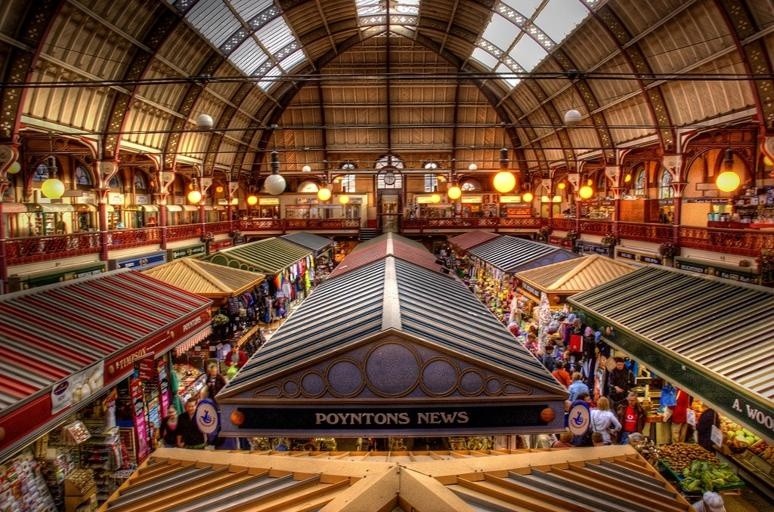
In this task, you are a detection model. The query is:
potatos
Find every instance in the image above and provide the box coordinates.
[652,442,720,473]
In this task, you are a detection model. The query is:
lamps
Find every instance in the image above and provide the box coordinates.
[41,153,65,200]
[432,177,440,202]
[557,171,631,198]
[247,190,257,205]
[564,81,583,128]
[196,82,214,129]
[492,161,517,194]
[264,132,287,196]
[524,176,533,202]
[317,180,331,200]
[189,172,201,204]
[303,150,312,173]
[447,180,462,199]
[298,180,317,193]
[716,147,741,193]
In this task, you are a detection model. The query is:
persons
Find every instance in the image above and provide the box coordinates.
[285,202,613,219]
[707,211,739,223]
[658,205,674,223]
[232,209,279,220]
[54,216,143,232]
[691,489,727,512]
[432,241,720,452]
[159,239,354,450]
[147,211,227,227]
